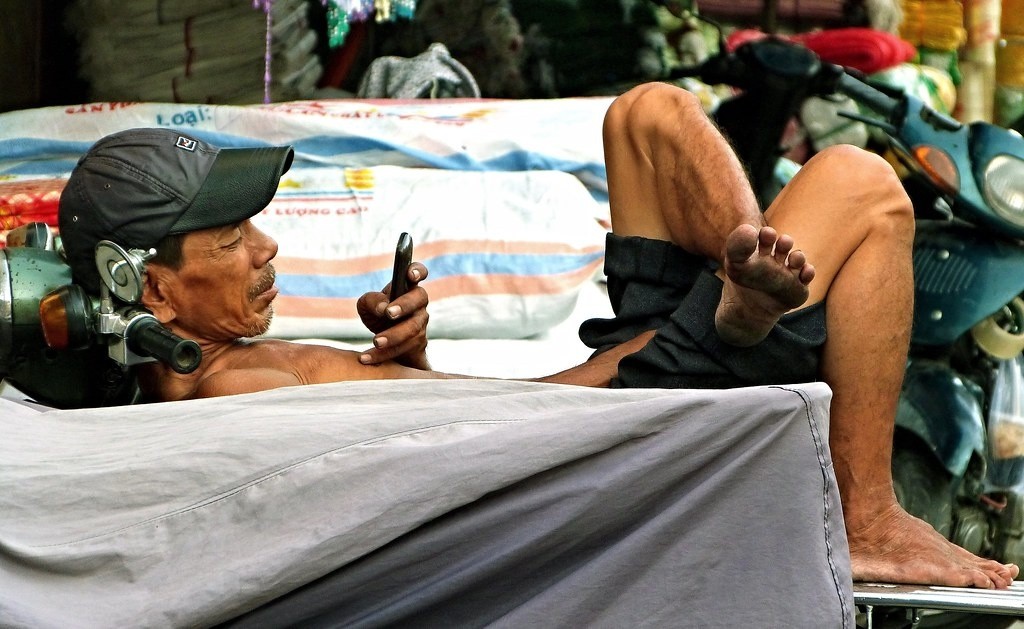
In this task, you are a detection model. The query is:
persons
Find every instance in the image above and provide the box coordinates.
[56,82,1020,590]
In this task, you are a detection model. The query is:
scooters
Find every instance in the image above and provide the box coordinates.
[647,1,1024,629]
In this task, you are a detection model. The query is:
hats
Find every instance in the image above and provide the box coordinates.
[57,127,294,285]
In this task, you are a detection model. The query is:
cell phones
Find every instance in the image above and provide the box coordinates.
[382,232,413,330]
